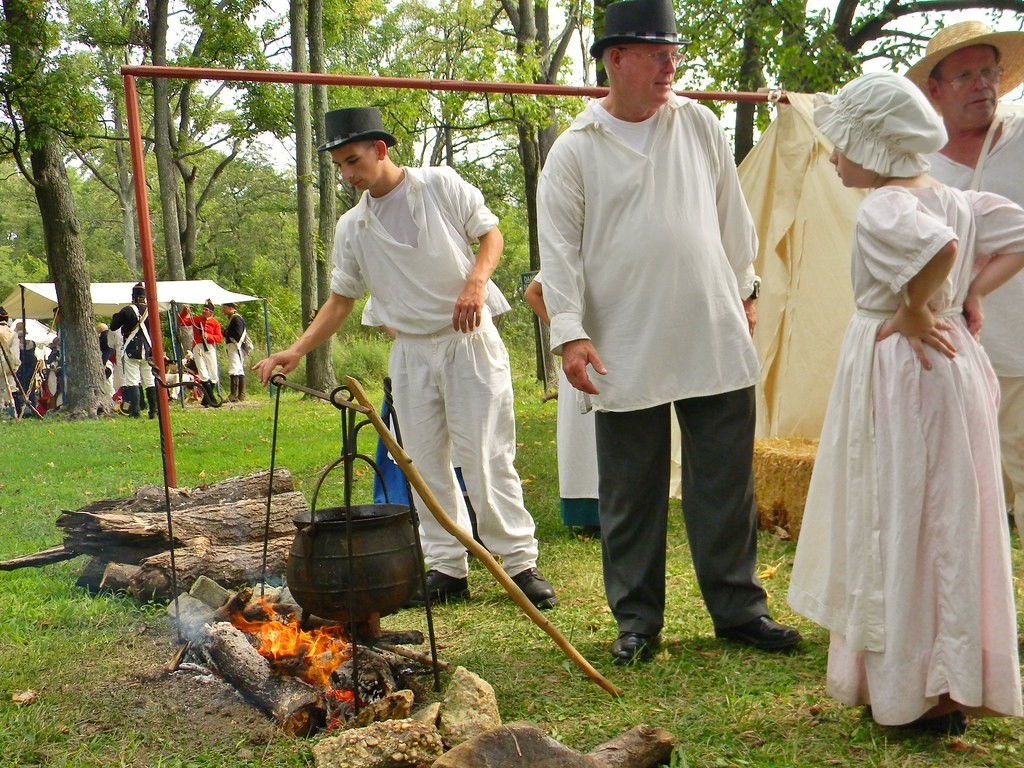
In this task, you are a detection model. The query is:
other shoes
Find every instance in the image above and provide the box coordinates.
[910,706,969,735]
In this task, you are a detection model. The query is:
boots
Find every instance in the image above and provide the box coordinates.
[126,386,141,419]
[238,375,245,401]
[145,386,158,419]
[200,381,218,407]
[222,374,238,402]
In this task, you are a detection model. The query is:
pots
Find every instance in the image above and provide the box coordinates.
[285,454,423,624]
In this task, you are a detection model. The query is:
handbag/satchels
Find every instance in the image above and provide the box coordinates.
[241,337,255,356]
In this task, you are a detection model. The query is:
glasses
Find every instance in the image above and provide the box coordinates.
[617,46,686,68]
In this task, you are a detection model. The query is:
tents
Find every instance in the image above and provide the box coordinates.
[0,279,273,416]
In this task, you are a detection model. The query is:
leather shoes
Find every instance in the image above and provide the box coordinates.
[610,630,661,668]
[406,567,468,605]
[713,614,801,649]
[512,568,556,609]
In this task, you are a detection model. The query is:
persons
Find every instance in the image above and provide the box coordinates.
[179,298,222,409]
[523,271,601,540]
[536,0,802,665]
[252,106,558,609]
[786,70,1024,739]
[97,281,159,419]
[0,307,60,409]
[902,20,1024,549]
[221,303,247,403]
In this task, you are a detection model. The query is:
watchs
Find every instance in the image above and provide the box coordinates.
[750,281,759,299]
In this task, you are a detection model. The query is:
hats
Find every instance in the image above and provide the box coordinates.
[133,282,146,297]
[813,68,948,178]
[0,306,9,322]
[590,0,692,58]
[903,22,1024,117]
[203,299,215,312]
[315,106,398,148]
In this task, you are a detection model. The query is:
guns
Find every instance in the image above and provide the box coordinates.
[0,342,44,420]
[0,356,20,425]
